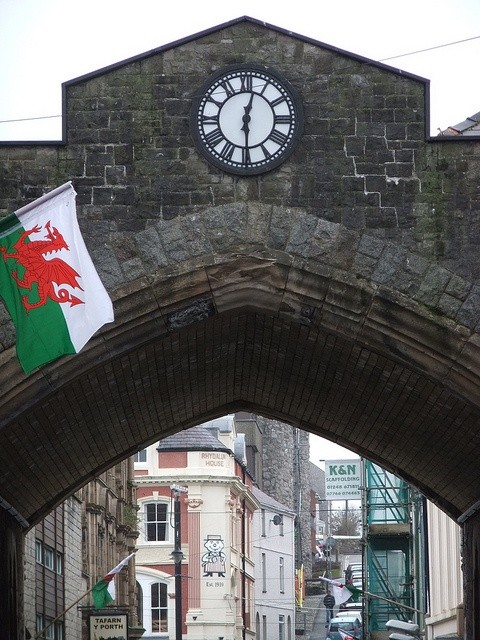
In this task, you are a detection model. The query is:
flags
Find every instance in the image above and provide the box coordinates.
[1,181,115,375]
[319,577,360,607]
[93,552,136,611]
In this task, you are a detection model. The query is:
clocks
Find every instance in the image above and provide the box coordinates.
[190,65,305,176]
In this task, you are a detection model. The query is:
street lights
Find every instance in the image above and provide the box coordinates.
[171,501,183,640]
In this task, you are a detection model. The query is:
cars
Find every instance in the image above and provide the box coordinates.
[326,617,363,640]
[339,562,370,611]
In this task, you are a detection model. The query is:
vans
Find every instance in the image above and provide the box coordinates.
[335,612,364,637]
